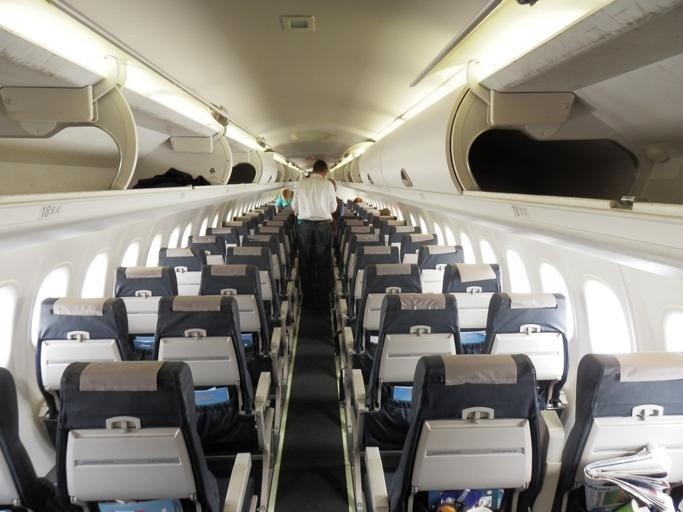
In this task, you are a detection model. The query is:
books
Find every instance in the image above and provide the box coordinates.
[195,388,228,405]
[135,337,156,342]
[133,340,155,360]
[98,499,175,511]
[429,488,505,512]
[461,332,486,344]
[392,387,413,402]
[241,331,254,346]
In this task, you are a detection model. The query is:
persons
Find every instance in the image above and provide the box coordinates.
[379,208,390,217]
[269,159,344,317]
[351,197,363,208]
